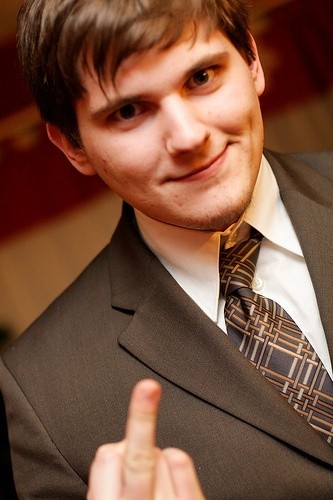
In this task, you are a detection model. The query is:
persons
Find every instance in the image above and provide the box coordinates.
[0,0,333,500]
